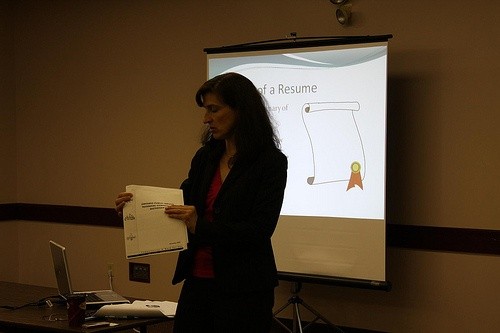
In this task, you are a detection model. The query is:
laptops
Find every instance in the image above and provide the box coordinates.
[49,240,131,307]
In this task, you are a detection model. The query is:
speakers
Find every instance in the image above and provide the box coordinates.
[327,0,348,8]
[335,8,352,25]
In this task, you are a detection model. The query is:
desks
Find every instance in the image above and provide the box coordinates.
[0,281,174,333]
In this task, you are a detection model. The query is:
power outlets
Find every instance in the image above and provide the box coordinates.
[129,262,150,283]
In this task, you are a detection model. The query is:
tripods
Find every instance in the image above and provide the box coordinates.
[272,296,340,333]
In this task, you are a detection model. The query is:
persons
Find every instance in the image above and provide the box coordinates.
[115,72,288,333]
